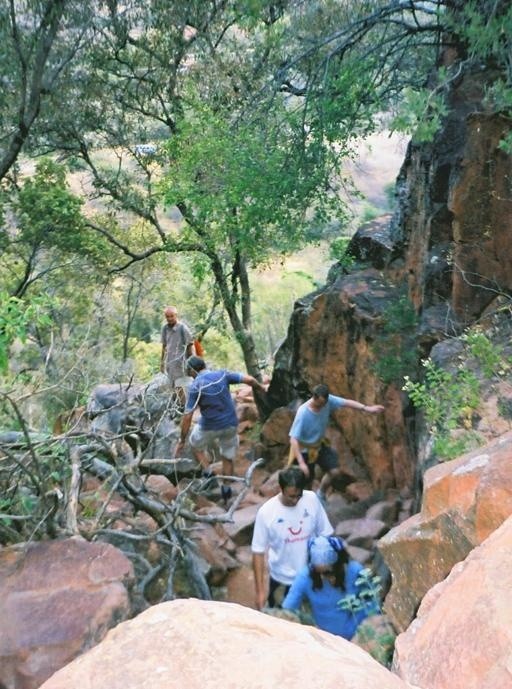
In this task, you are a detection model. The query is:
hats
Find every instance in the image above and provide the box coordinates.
[188,355,206,376]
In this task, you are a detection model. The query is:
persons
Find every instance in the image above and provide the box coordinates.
[174,355,270,485]
[282,536,384,640]
[159,306,196,411]
[251,467,334,610]
[288,384,384,507]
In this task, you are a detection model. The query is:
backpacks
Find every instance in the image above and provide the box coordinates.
[186,338,208,381]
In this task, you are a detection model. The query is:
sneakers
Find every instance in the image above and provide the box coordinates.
[204,469,232,499]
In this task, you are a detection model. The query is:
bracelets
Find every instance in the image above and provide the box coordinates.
[178,438,185,443]
[360,404,368,411]
